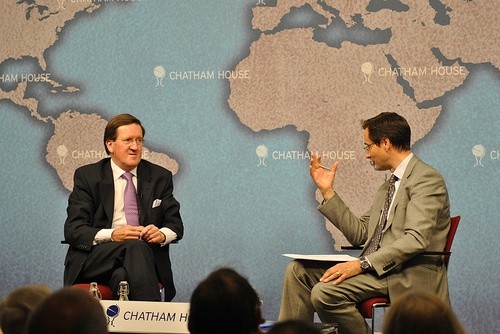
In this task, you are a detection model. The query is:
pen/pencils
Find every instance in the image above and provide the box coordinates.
[312,161,331,171]
[260,325,271,328]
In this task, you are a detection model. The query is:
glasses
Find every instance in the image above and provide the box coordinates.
[114,138,146,145]
[363,142,374,151]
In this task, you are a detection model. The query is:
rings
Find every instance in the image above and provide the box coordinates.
[336,271,340,276]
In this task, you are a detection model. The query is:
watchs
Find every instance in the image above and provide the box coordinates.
[359,257,370,273]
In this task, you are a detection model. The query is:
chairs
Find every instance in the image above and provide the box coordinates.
[340,216,461,334]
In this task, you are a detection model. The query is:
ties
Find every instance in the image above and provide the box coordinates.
[363,174,398,256]
[120,173,139,226]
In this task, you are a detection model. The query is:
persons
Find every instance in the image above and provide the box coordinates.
[0,269,437,334]
[62,113,184,302]
[278,112,451,334]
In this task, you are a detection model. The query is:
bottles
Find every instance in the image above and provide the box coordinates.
[89,282,99,300]
[119,281,129,301]
